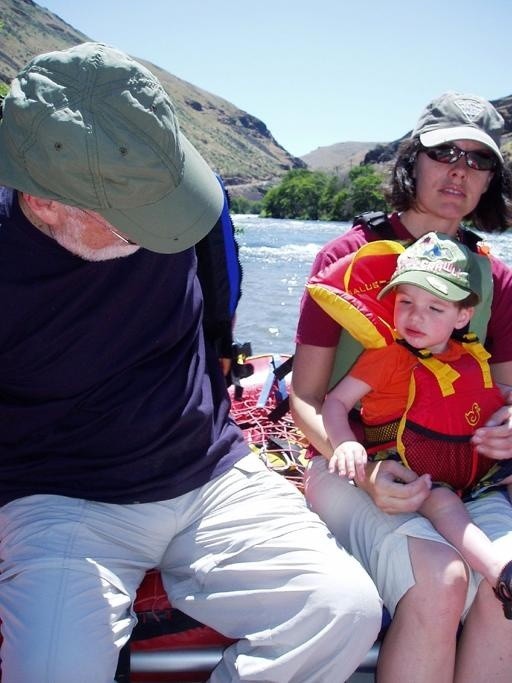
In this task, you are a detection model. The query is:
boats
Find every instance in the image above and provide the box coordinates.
[1,353,510,683]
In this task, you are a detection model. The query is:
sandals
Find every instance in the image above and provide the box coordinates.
[492,560,512,619]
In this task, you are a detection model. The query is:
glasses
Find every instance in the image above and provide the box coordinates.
[424,144,497,170]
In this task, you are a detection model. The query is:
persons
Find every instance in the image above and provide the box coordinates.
[320,229,512,621]
[289,90,512,683]
[0,39,385,683]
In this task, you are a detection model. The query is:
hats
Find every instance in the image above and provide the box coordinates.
[411,92,505,165]
[0,42,224,255]
[377,230,482,303]
